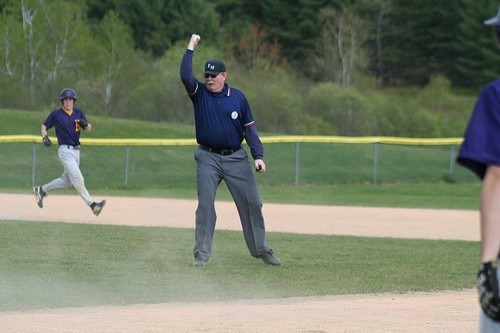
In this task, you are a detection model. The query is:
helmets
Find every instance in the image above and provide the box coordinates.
[60,89,76,100]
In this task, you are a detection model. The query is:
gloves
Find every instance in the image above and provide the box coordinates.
[77,119,88,130]
[43,136,51,146]
[475,260,500,325]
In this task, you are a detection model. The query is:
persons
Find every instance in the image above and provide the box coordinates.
[455,11,500,332]
[32,89,105,217]
[180,33,282,267]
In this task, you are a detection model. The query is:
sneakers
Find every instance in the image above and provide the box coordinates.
[261,248,281,266]
[92,199,105,216]
[32,186,45,208]
[193,254,209,266]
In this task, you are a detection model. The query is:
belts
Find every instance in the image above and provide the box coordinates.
[68,145,78,149]
[200,144,243,155]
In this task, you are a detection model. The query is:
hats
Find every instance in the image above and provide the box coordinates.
[204,59,225,76]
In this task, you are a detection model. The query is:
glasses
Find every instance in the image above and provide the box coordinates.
[204,73,218,78]
